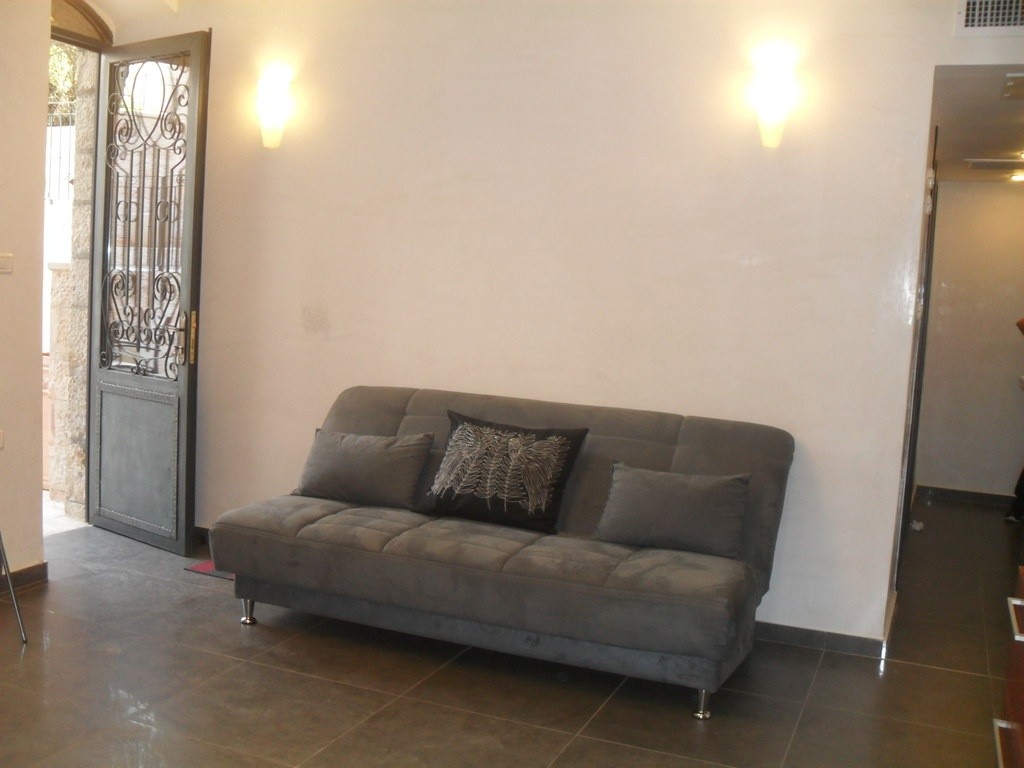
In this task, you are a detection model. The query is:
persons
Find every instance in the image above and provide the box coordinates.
[1004,318,1024,529]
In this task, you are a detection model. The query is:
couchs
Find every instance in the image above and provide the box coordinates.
[206,388,796,724]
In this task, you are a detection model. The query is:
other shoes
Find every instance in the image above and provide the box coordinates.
[1005,498,1024,524]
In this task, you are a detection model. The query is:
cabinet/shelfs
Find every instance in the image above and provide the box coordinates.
[989,563,1024,768]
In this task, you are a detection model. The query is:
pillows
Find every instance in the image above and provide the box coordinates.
[589,463,755,558]
[290,430,433,512]
[428,410,590,536]
